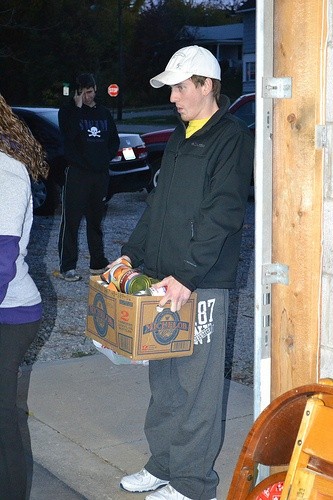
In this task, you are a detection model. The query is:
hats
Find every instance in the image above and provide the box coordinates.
[149,45,221,89]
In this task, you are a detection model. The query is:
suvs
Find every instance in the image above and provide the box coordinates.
[140,92,255,196]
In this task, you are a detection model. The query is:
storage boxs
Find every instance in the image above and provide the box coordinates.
[84,275,197,362]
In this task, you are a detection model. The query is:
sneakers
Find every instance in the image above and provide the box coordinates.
[59,269,82,281]
[119,468,171,492]
[90,268,104,274]
[145,483,216,500]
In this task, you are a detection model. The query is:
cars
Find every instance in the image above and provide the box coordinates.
[9,106,152,213]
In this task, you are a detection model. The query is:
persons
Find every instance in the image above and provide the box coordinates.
[0,93,50,500]
[58,72,121,282]
[107,45,256,500]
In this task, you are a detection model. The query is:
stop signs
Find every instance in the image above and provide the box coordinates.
[108,83,119,97]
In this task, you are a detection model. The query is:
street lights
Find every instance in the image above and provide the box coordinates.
[89,0,124,120]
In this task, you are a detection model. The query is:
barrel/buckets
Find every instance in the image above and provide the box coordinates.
[108,264,151,295]
